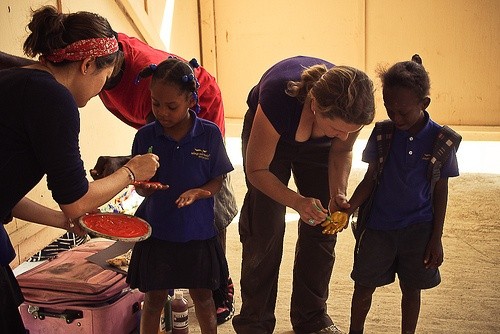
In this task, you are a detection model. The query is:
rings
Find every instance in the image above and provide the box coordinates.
[308,218,315,224]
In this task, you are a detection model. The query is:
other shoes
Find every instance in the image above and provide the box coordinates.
[316,325,348,334]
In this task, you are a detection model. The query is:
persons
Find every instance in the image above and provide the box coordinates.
[232,55,375,334]
[132,55,233,334]
[0,6,160,334]
[322,53,462,334]
[99,32,235,325]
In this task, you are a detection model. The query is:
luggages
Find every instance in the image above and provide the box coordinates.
[16,238,145,334]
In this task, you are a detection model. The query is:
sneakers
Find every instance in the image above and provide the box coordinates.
[215,277,235,325]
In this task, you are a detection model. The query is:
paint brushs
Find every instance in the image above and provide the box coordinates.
[312,202,338,228]
[148,146,152,153]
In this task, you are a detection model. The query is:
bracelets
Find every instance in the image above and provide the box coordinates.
[122,166,135,185]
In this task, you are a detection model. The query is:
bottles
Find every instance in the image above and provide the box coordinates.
[160,288,189,334]
